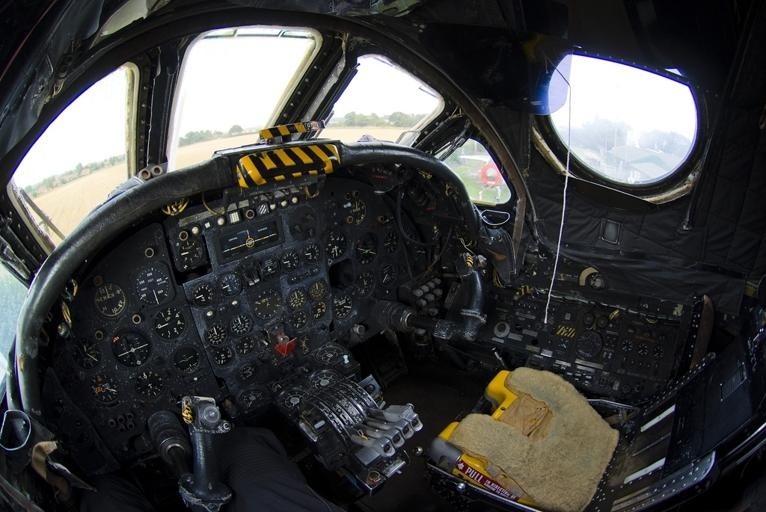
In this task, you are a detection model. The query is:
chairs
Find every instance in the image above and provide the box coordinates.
[420,323,766,512]
